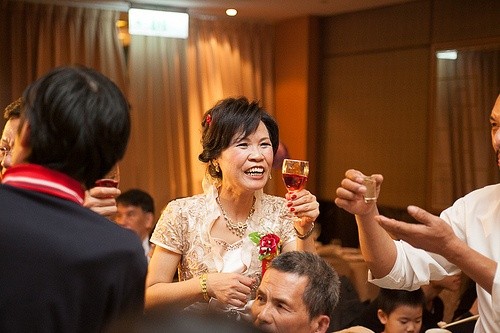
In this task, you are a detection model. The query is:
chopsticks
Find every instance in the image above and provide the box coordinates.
[442,314,479,328]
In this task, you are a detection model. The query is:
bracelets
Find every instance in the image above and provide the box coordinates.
[294,221,315,239]
[216,193,257,238]
[198,274,210,302]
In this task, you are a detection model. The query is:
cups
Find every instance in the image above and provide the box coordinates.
[362,176,376,203]
[90,163,119,188]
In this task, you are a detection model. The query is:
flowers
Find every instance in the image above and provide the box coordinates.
[249,229,282,264]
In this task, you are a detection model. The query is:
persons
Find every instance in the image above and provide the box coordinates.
[375,288,424,333]
[143,94,320,322]
[334,92,500,333]
[250,251,341,333]
[0,96,123,224]
[342,270,461,333]
[0,61,149,333]
[116,189,154,265]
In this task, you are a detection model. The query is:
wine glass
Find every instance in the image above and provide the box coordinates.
[280,159,309,221]
